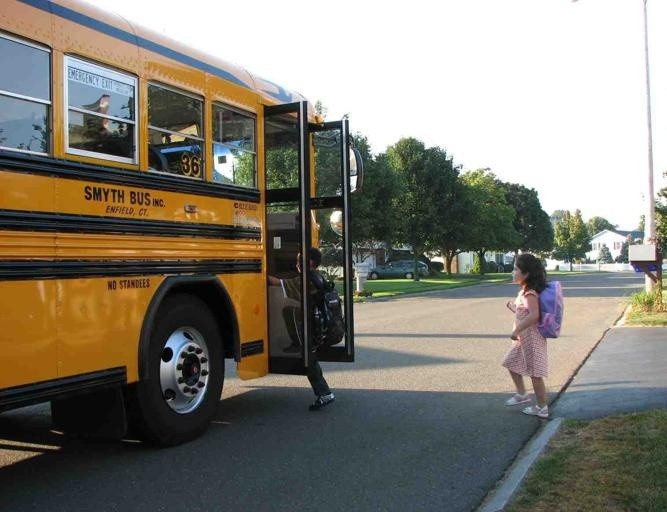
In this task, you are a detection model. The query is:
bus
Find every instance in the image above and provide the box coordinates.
[0,1,366,446]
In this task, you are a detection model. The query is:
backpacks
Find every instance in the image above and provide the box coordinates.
[308,273,343,346]
[537,281,563,337]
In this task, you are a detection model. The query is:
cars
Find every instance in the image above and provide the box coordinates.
[368,259,430,279]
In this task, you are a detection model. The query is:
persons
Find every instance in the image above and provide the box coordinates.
[503,253,551,419]
[268,247,338,412]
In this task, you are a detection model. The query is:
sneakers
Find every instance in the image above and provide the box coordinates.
[505,393,529,406]
[309,393,336,409]
[282,344,303,353]
[523,404,549,418]
